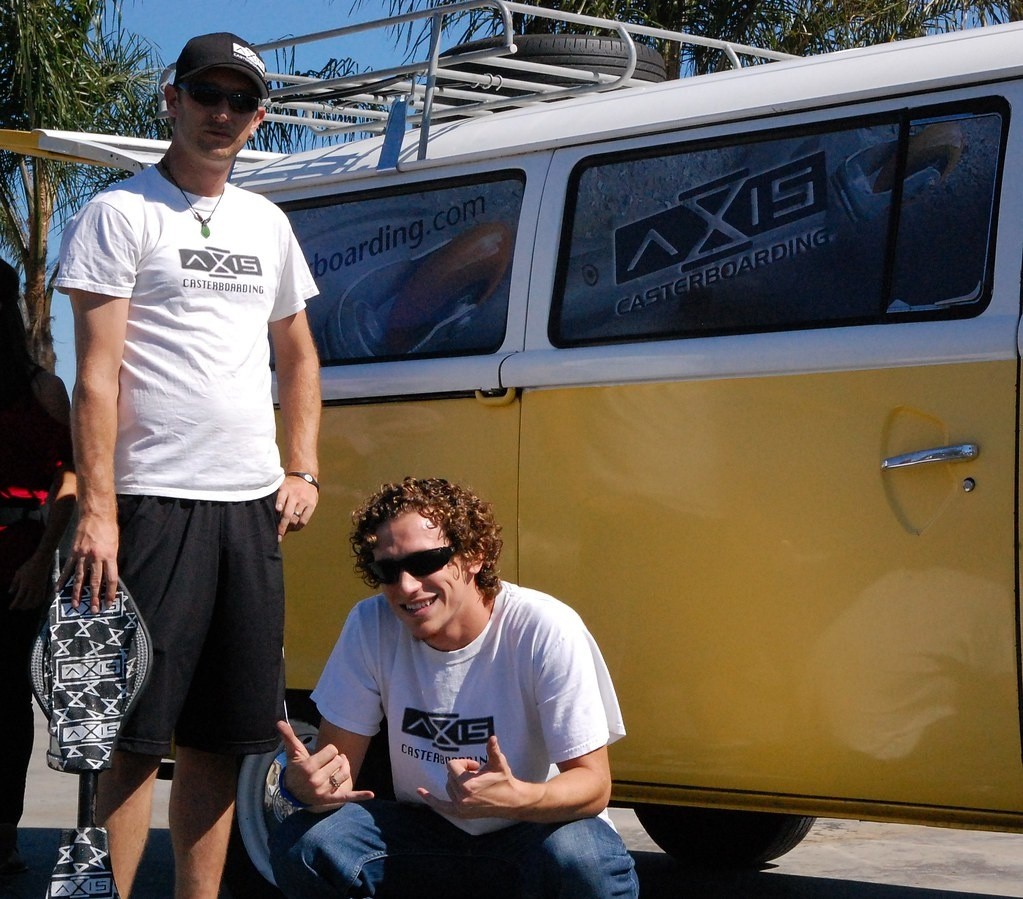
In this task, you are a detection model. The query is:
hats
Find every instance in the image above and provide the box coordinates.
[168,32,270,100]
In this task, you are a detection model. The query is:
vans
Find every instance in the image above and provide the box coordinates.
[0,1,1023,898]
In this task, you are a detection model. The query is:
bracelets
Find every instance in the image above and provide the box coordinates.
[279,766,313,809]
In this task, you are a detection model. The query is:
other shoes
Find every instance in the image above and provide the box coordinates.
[0,823,25,876]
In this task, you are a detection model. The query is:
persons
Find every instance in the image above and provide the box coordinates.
[266,477,639,899]
[0,257,77,873]
[51,33,321,899]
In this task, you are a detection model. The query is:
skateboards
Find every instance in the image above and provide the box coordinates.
[27,568,153,899]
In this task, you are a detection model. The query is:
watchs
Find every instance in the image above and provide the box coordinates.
[286,472,319,494]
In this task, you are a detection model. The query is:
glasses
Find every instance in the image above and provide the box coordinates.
[177,82,263,114]
[367,543,457,585]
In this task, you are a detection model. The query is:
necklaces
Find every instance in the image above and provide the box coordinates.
[161,158,225,238]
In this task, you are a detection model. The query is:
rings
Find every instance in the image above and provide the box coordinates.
[330,774,340,789]
[293,511,301,518]
